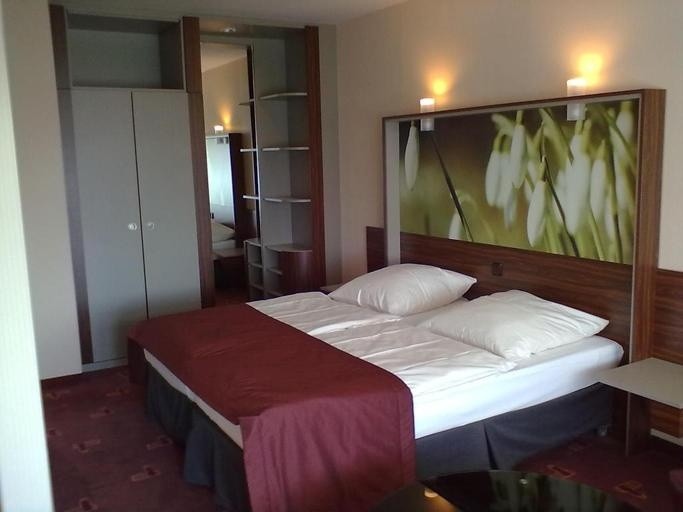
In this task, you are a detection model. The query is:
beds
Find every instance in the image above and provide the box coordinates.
[133,291,627,512]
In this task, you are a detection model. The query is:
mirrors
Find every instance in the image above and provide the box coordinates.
[200,40,265,306]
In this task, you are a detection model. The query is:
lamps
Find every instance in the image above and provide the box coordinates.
[566,79,587,121]
[420,97,436,132]
[214,125,225,145]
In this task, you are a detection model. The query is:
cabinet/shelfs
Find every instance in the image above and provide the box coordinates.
[266,243,315,297]
[244,238,265,300]
[69,84,202,374]
[257,92,310,203]
[237,98,258,201]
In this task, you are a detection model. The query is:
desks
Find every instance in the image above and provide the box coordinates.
[368,469,641,512]
[597,356,682,455]
[213,248,246,287]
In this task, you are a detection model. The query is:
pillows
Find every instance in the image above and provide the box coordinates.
[210,219,235,243]
[326,263,611,360]
